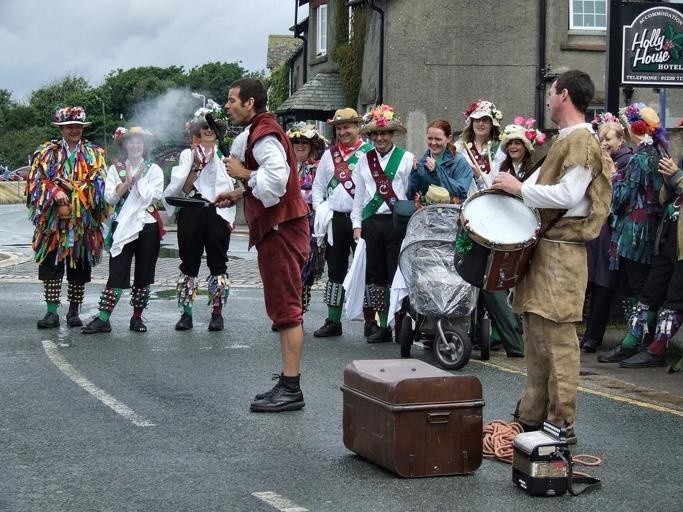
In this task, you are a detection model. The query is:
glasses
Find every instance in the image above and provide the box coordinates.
[202,124,213,130]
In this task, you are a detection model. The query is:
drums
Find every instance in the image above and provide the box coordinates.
[455,188,541,290]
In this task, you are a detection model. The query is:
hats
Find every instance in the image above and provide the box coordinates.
[327,108,362,126]
[51,107,92,128]
[619,103,663,143]
[465,101,503,126]
[415,185,461,209]
[501,124,534,153]
[358,104,407,137]
[116,127,153,145]
[286,122,325,157]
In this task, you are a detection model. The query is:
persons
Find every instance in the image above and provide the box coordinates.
[312,107,374,335]
[272,121,329,330]
[225,78,312,413]
[350,105,407,343]
[80,127,164,333]
[583,101,683,368]
[25,106,113,329]
[489,70,612,442]
[164,107,237,330]
[454,100,506,192]
[407,119,472,203]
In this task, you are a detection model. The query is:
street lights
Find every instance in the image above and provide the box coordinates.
[191,93,205,108]
[98,100,107,165]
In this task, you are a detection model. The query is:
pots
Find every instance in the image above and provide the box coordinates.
[165,197,233,209]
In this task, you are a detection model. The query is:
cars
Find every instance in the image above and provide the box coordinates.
[0,166,32,181]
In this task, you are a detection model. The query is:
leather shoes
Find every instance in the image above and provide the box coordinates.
[130,317,146,332]
[582,340,665,367]
[314,320,393,342]
[37,313,112,333]
[176,313,193,329]
[250,375,305,412]
[209,313,224,331]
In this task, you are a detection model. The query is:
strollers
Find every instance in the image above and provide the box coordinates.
[400,203,490,369]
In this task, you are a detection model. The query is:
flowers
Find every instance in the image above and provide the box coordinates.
[465,102,503,120]
[505,116,546,145]
[591,112,620,127]
[111,126,153,141]
[55,106,86,122]
[362,104,403,127]
[285,121,329,148]
[186,99,229,129]
[625,103,659,135]
